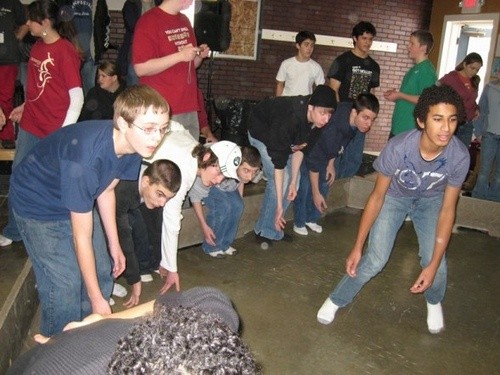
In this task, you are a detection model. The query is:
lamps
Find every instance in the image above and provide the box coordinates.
[458,0,486,13]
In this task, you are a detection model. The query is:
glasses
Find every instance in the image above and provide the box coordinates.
[128,120,171,134]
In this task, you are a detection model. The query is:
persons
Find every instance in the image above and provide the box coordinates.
[76,60,127,123]
[274,30,325,96]
[189,141,262,258]
[470,57,500,203]
[326,20,380,102]
[193,84,218,145]
[383,31,438,142]
[247,85,338,249]
[69,0,111,100]
[138,119,242,294]
[10,84,172,340]
[0,286,263,375]
[317,84,470,336]
[0,0,84,246]
[0,108,7,131]
[96,158,181,308]
[293,92,379,235]
[132,0,211,143]
[436,52,482,195]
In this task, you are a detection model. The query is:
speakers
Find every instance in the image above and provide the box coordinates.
[194,0,233,51]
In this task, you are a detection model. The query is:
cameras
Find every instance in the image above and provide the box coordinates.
[194,47,203,54]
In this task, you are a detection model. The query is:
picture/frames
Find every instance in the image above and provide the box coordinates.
[207,0,265,63]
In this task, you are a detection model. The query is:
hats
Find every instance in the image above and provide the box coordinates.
[308,84,338,109]
[210,141,243,181]
